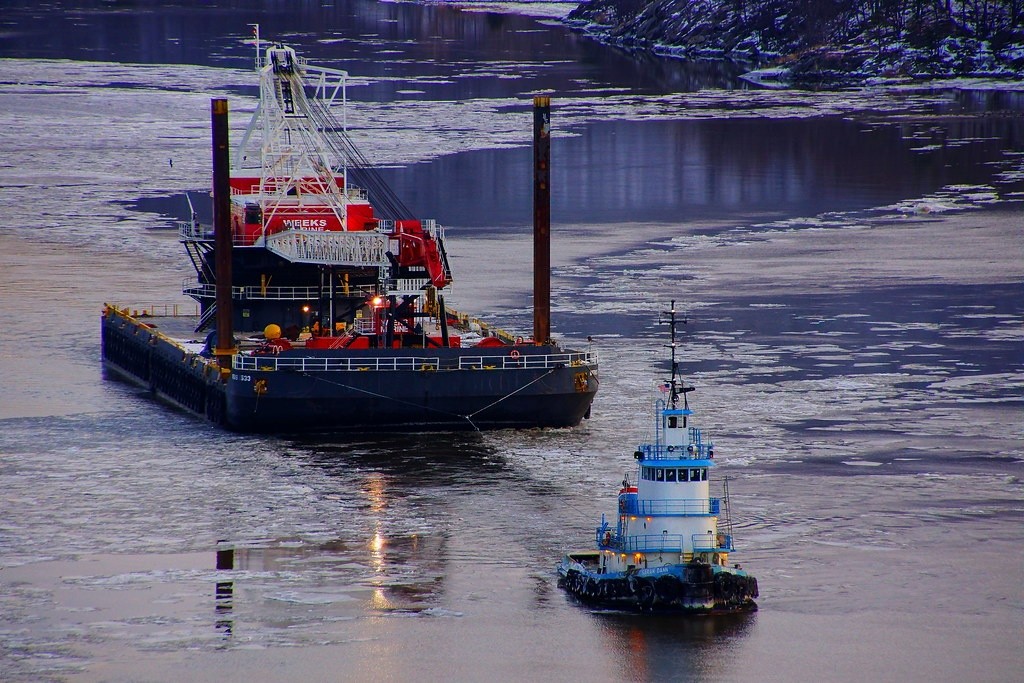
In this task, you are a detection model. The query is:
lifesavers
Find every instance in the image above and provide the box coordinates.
[510,349,519,359]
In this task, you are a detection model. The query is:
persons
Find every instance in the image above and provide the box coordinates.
[252,324,292,354]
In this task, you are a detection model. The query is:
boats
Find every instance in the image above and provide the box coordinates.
[92,91,605,442]
[556,296,761,619]
[178,30,453,306]
